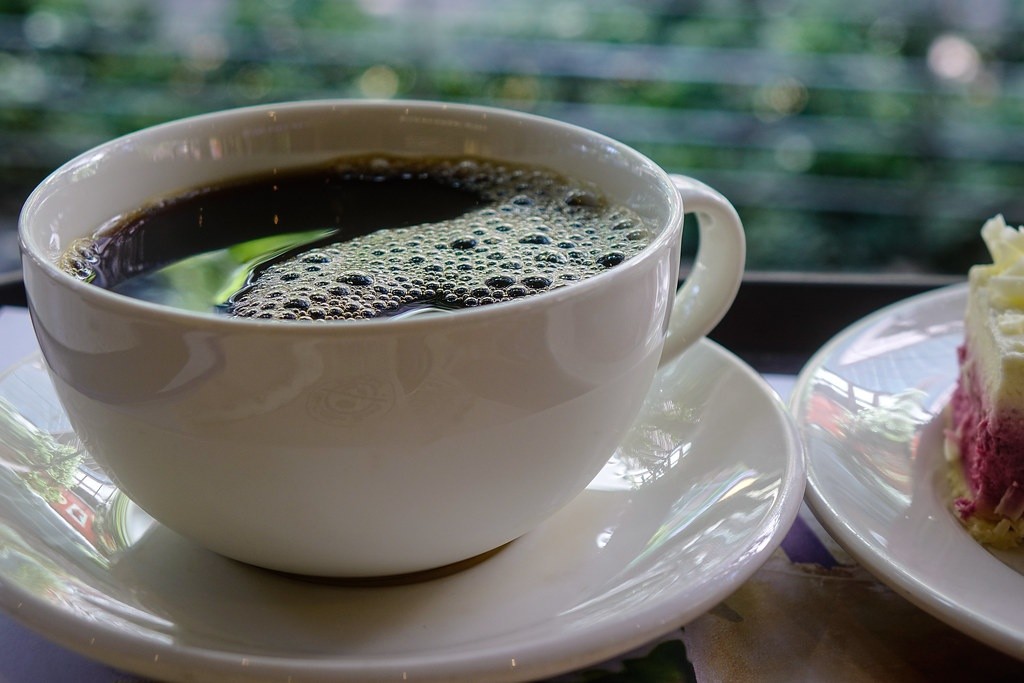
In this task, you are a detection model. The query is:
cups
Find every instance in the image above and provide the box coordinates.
[18,94,750,585]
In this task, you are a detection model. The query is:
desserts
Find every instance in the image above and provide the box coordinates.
[944,213,1024,554]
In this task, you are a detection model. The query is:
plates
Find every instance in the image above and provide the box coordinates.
[0,321,807,683]
[786,280,1024,658]
